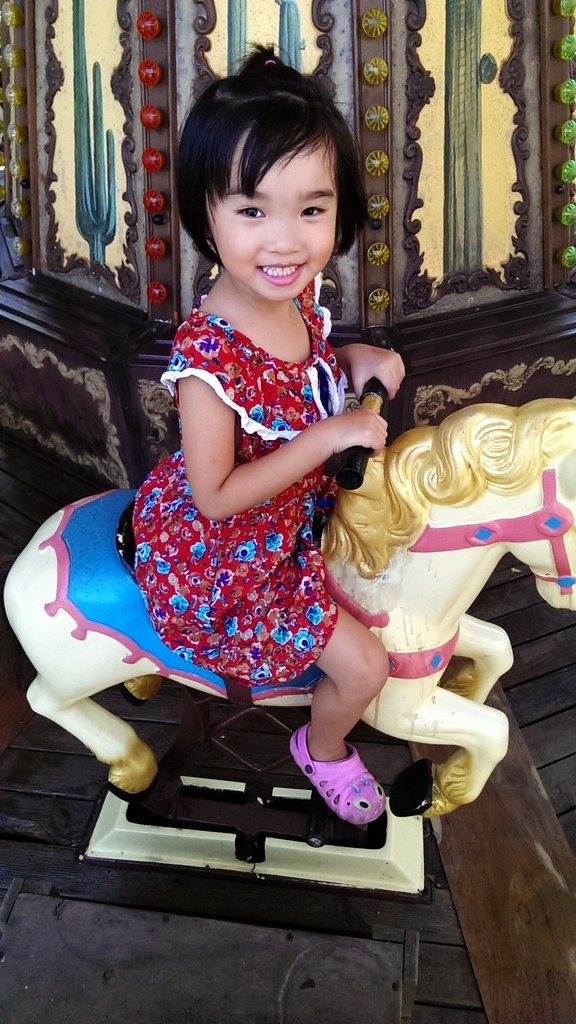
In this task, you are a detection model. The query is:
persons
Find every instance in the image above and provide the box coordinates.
[134,44,405,825]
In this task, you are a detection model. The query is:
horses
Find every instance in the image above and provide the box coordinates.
[5,396,574,821]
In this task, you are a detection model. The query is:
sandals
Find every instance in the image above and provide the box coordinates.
[289,722,386,825]
